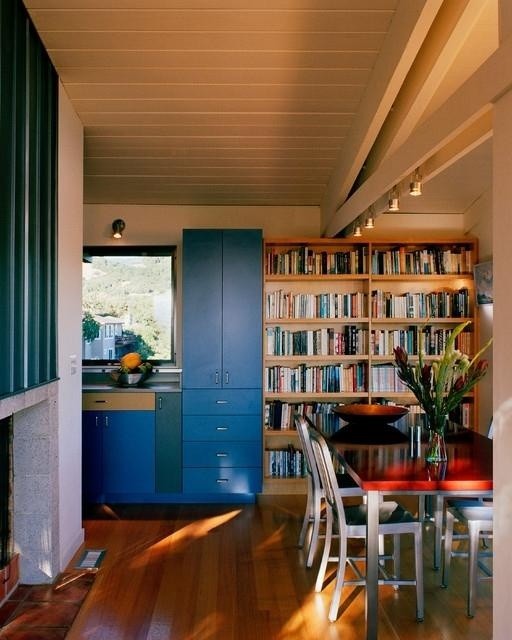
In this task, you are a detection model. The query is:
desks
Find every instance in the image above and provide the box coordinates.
[303,413,493,640]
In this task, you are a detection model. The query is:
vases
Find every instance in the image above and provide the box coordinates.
[118,373,143,387]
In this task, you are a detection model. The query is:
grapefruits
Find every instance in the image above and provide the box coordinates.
[120,353,143,368]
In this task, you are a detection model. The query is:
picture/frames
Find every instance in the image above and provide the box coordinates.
[472,259,494,308]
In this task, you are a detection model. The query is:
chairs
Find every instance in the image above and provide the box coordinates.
[306,427,425,624]
[441,499,494,619]
[431,411,495,571]
[292,412,387,569]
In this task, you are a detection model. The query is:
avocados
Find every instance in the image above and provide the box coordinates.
[117,362,152,374]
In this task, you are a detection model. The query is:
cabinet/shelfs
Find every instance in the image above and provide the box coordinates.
[263,235,479,497]
[182,228,262,505]
[82,393,182,505]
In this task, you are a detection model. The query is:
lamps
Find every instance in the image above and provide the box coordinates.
[351,203,375,238]
[379,167,424,212]
[111,220,125,239]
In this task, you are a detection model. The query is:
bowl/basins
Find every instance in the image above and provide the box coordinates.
[331,403,409,425]
[328,424,413,445]
[109,372,156,386]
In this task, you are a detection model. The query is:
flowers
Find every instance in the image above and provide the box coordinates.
[390,314,494,456]
[108,352,154,383]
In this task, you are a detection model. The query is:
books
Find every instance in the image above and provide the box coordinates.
[373,398,471,438]
[266,324,368,394]
[370,326,472,392]
[265,399,356,480]
[371,248,472,318]
[266,247,366,319]
